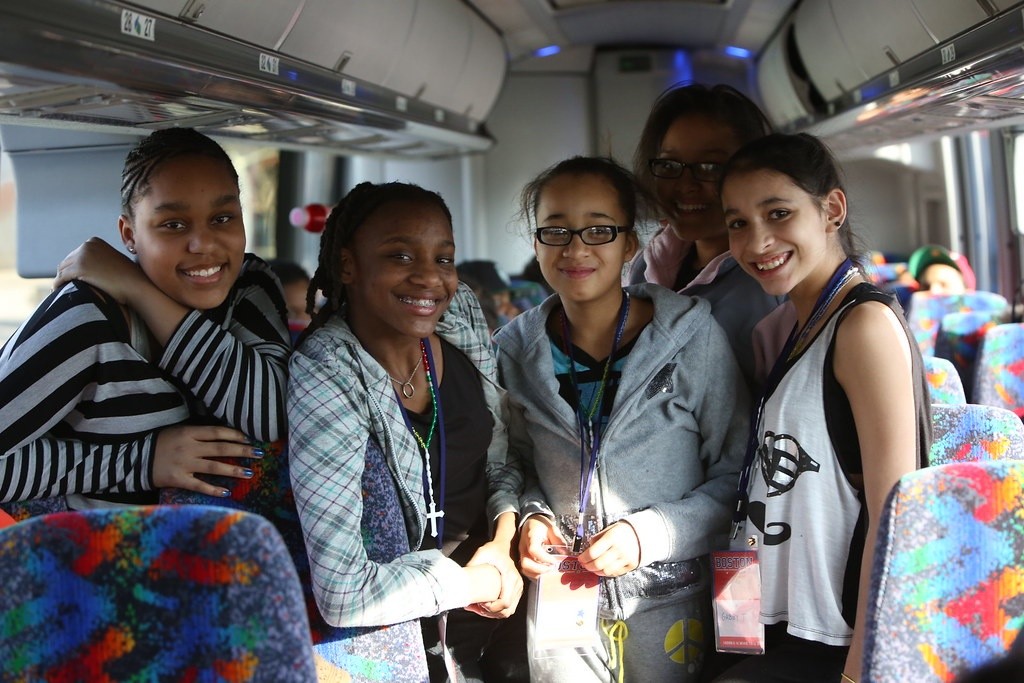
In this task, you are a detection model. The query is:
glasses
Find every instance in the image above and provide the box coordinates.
[534,226,629,246]
[646,157,725,183]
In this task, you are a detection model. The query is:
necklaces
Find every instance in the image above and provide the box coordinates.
[787,267,859,360]
[390,339,445,538]
[558,289,631,450]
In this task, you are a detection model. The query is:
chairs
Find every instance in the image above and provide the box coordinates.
[0,251,1024,682]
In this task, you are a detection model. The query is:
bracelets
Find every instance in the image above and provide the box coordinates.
[841,673,855,683]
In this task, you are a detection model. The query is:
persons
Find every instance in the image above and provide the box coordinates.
[1,125,310,504]
[284,181,525,683]
[491,157,751,683]
[909,243,965,291]
[621,81,935,683]
